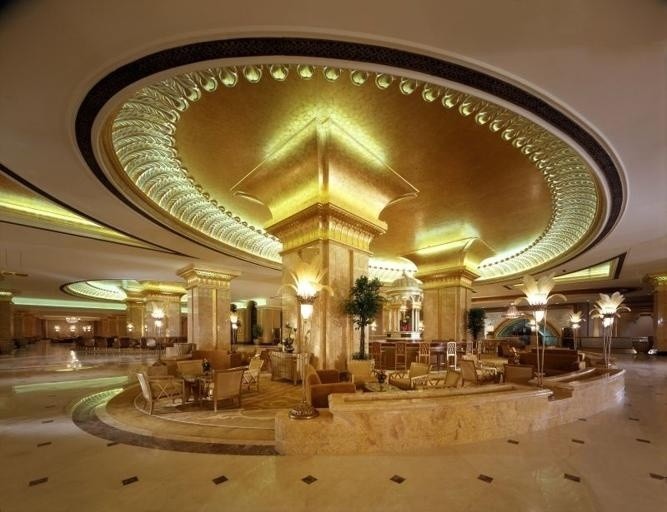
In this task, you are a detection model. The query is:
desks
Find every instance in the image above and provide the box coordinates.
[268,351,310,386]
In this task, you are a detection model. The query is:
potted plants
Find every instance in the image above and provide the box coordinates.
[344,273,387,386]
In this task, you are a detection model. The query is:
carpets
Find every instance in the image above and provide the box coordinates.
[105,372,332,441]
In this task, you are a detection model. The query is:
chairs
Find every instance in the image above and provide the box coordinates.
[302,341,535,407]
[134,356,265,416]
[76,336,177,352]
